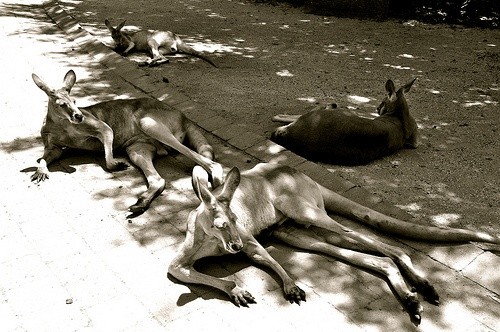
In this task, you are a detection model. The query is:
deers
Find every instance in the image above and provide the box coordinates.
[31,70,225,213]
[105,19,217,68]
[270,78,418,166]
[168,160,442,327]
